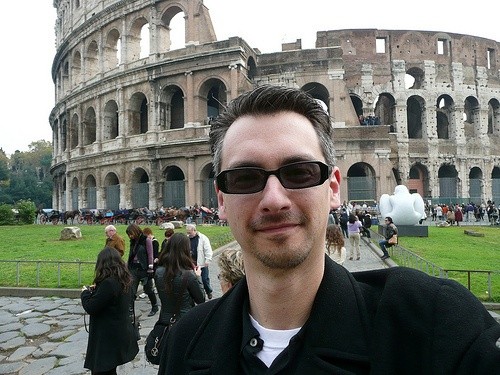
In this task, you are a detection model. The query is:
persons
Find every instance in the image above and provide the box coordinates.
[345,213,363,260]
[141,84,500,375]
[79,245,141,375]
[378,216,399,260]
[328,192,500,228]
[36,200,228,225]
[158,231,205,325]
[102,225,213,316]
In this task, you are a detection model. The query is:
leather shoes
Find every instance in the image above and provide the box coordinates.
[148,305,159,317]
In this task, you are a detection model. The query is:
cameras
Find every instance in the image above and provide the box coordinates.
[86,285,96,292]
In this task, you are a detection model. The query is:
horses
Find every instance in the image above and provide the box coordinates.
[64,208,81,224]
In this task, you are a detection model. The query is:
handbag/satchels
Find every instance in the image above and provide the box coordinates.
[387,234,397,243]
[144,319,176,364]
[132,320,142,340]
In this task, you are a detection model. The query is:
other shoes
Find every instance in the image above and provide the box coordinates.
[356,256,360,260]
[381,255,390,260]
[208,292,212,299]
[349,256,353,260]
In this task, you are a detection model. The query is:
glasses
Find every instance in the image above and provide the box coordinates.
[214,160,333,194]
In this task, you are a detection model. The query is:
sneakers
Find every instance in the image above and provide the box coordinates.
[154,286,158,294]
[138,293,148,298]
